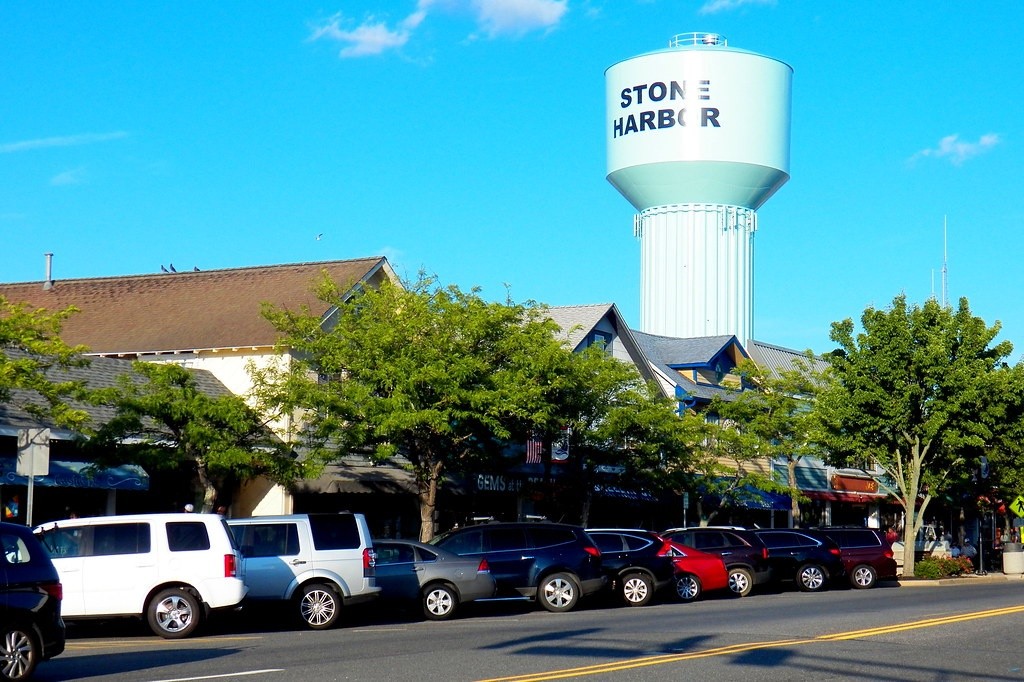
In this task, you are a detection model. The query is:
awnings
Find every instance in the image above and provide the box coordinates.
[0,459,151,489]
[575,464,793,511]
[265,464,467,496]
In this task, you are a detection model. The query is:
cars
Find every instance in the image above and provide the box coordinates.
[0,521,66,682]
[369,538,498,621]
[650,531,730,603]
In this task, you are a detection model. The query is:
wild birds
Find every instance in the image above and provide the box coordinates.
[160,264,176,272]
[316,233,323,240]
[194,266,200,271]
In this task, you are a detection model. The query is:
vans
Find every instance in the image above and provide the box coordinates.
[15,513,251,640]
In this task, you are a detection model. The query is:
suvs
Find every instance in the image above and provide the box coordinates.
[806,527,898,589]
[655,524,773,598]
[391,519,609,613]
[206,509,384,630]
[748,528,847,592]
[583,528,676,607]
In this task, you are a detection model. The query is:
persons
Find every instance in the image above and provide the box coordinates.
[886,516,1020,573]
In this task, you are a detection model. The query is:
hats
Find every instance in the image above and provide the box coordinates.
[184,504,195,514]
[964,538,971,544]
